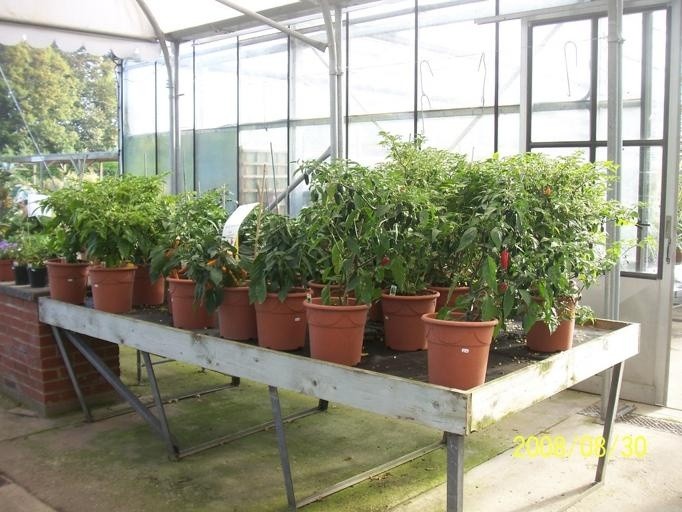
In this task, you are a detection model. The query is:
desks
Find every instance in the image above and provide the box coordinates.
[40,293,640,512]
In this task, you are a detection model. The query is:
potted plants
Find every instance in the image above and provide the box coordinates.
[0,130,656,391]
